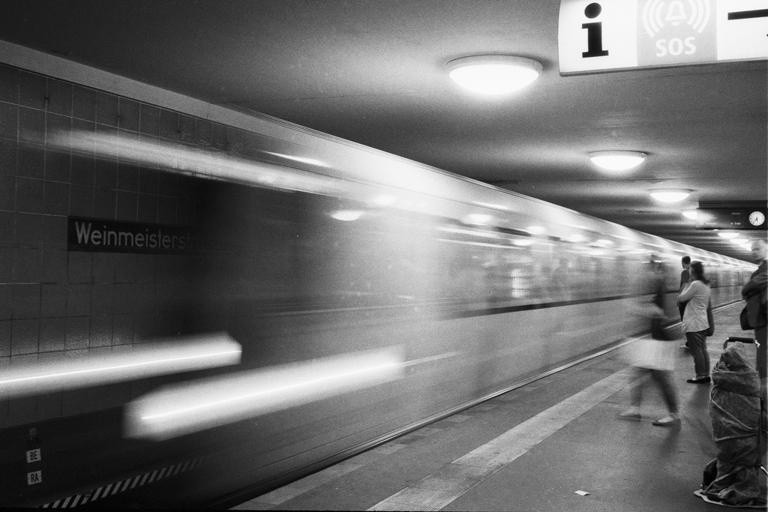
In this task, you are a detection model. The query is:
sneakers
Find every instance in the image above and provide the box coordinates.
[616,410,642,421]
[680,345,692,350]
[651,414,681,427]
[687,376,711,384]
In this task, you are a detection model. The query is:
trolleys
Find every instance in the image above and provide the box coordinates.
[687,336,768,509]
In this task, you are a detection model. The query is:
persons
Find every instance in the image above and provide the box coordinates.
[468,250,575,305]
[676,256,692,349]
[621,249,679,427]
[739,238,768,386]
[675,260,713,386]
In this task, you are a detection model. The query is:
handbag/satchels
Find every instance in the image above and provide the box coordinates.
[651,322,687,340]
[704,307,714,336]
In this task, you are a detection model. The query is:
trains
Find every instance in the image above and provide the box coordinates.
[1,86,768,512]
